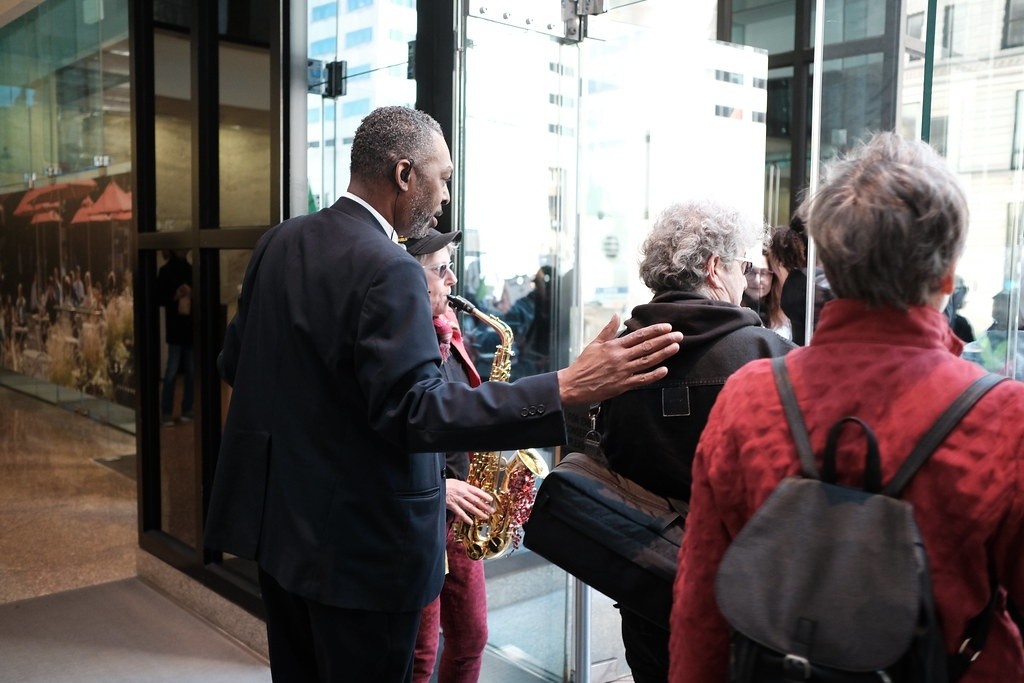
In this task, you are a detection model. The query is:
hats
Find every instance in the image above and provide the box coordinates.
[403,227,462,257]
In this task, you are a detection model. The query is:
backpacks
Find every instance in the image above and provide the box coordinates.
[714,357,1010,683]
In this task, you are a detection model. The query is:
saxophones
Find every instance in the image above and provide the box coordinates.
[449,292,551,563]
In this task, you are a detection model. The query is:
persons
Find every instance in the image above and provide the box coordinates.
[599,198,832,683]
[157,246,193,425]
[667,133,1024,683]
[964,289,1024,381]
[202,106,683,683]
[17,266,116,340]
[396,227,496,683]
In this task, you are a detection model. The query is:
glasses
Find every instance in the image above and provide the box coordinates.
[736,257,753,276]
[422,262,455,279]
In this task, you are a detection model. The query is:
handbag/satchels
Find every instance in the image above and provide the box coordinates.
[521,429,690,634]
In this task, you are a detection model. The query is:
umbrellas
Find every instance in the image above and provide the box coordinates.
[13,176,132,275]
